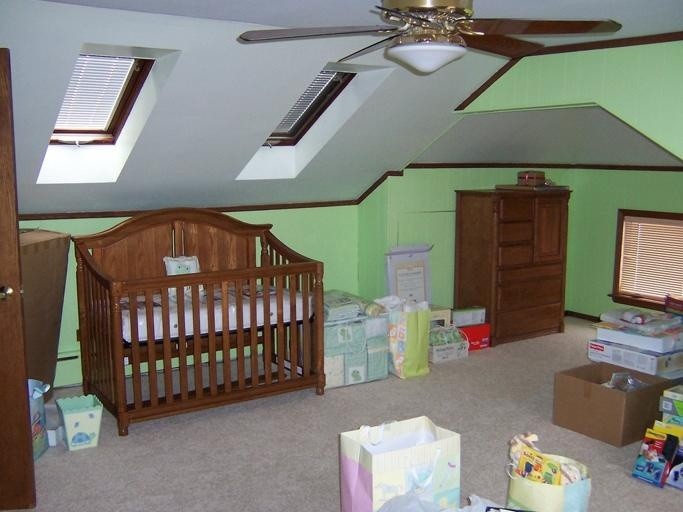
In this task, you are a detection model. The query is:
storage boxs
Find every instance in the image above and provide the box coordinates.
[554,309,683,447]
[28,378,103,461]
[324,289,490,390]
[18,227,72,404]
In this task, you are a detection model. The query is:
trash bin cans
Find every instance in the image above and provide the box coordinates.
[54,393,104,452]
[27,379,49,461]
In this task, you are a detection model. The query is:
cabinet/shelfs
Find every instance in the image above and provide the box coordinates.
[455,188,570,347]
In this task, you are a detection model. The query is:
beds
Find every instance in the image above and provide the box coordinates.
[72,207,326,437]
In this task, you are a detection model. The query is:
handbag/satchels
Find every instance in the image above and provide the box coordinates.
[506,452,592,512]
[339,416,460,511]
[388,307,429,380]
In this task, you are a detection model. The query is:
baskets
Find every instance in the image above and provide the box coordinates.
[429,326,469,364]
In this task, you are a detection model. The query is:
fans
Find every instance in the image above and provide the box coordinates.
[239,0,620,76]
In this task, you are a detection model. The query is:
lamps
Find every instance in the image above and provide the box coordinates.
[382,1,474,78]
